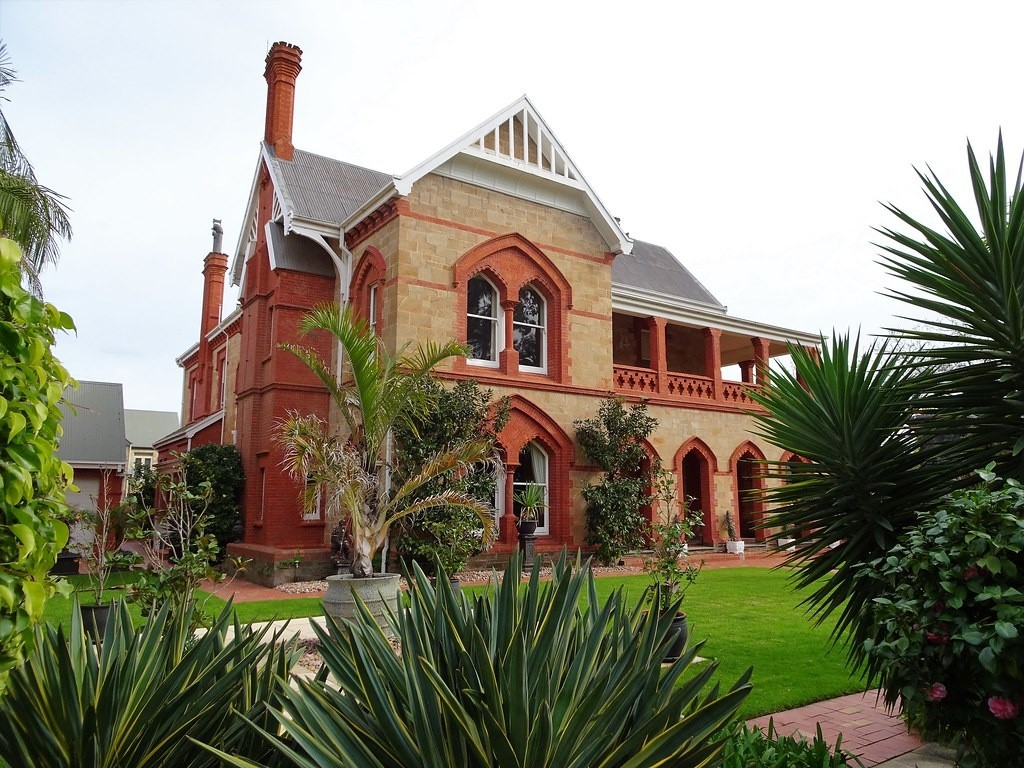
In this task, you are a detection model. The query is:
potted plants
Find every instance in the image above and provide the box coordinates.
[49,513,82,575]
[270,303,498,648]
[722,511,744,554]
[67,464,128,645]
[508,481,550,534]
[778,525,796,551]
[671,515,688,557]
